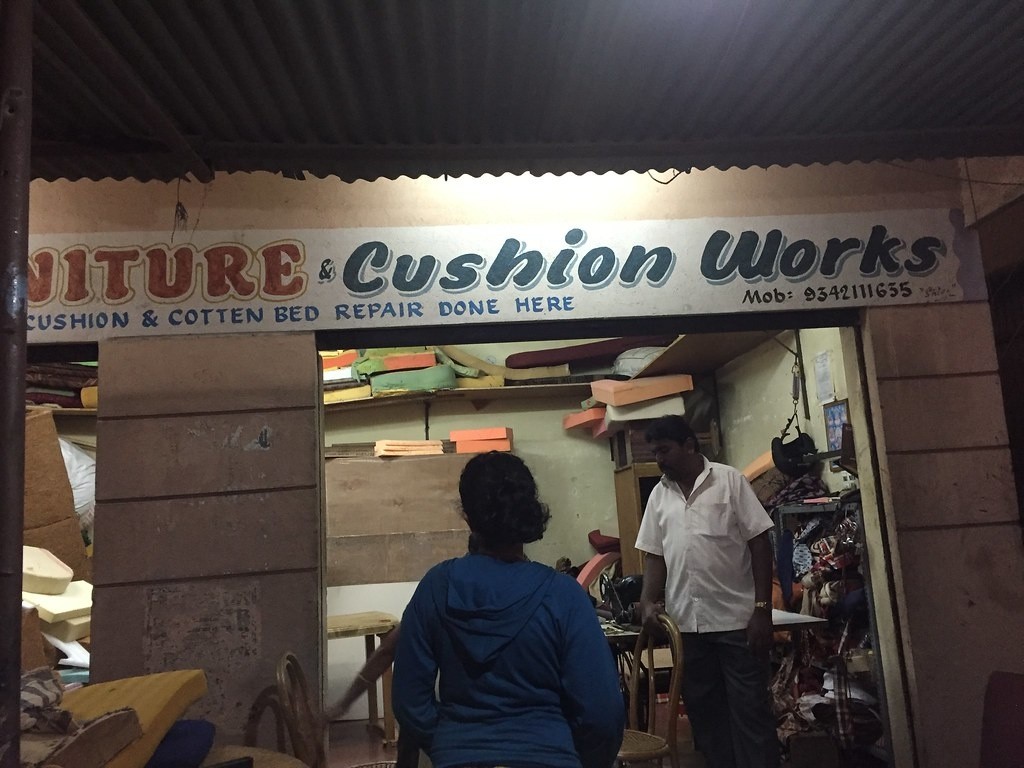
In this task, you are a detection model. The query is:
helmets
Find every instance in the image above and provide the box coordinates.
[771,432,816,477]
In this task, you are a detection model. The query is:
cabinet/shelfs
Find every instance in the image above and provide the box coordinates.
[613,461,663,576]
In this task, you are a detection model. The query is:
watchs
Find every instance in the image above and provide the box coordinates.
[754,601,773,610]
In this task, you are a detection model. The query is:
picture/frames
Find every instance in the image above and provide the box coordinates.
[822,398,851,473]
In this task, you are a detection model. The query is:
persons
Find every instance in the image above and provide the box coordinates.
[635,413,784,768]
[390,450,626,768]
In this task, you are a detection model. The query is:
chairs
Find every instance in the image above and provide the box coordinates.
[276,651,397,768]
[616,612,684,768]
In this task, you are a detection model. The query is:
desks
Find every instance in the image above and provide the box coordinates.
[327,611,399,749]
[600,610,828,653]
